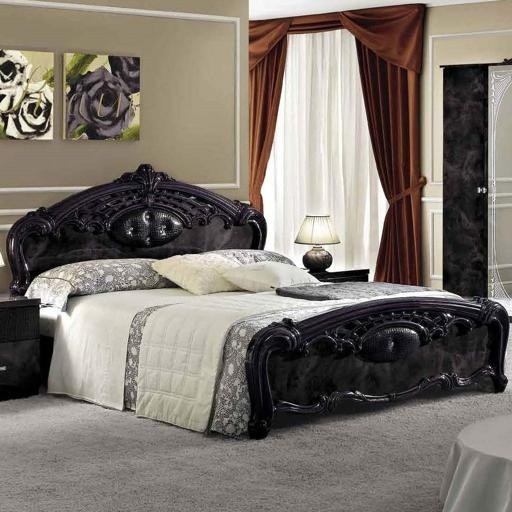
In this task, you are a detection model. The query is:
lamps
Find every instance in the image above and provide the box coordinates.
[294,215,341,273]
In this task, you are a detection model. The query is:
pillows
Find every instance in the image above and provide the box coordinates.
[24,249,321,312]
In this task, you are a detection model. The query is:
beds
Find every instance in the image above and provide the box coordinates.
[6,164,510,439]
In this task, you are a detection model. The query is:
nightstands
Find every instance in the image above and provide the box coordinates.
[0,294,41,402]
[300,268,370,282]
